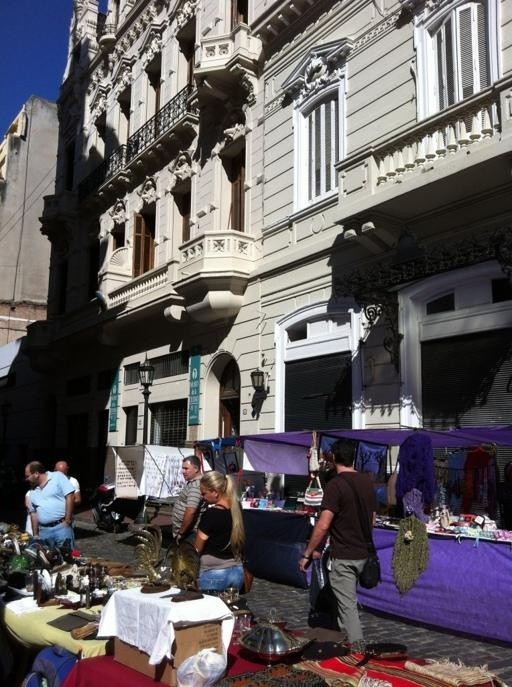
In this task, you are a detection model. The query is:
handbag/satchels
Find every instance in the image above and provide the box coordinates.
[359,555,382,589]
[239,568,253,595]
[303,432,325,507]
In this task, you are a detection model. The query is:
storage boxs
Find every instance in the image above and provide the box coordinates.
[113,620,222,687]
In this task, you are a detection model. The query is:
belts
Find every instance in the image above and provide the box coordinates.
[38,517,64,527]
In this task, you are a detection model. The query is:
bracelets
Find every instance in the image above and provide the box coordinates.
[178,531,184,537]
[301,553,310,559]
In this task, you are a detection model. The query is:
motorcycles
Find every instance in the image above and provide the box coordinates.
[92,479,159,530]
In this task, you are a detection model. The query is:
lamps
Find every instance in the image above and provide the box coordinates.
[251,367,270,394]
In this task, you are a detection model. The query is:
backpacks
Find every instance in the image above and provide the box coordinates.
[21,645,79,687]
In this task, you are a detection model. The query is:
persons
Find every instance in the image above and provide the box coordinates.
[24,485,36,535]
[53,459,82,549]
[23,460,76,550]
[193,470,248,596]
[170,454,207,579]
[297,437,378,645]
